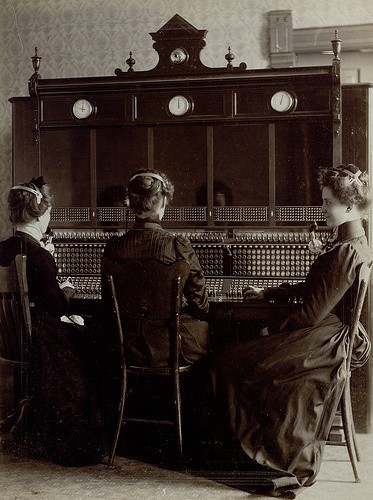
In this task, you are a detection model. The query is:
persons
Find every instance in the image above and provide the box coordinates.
[207,164,373,500]
[101,168,224,452]
[0,170,111,468]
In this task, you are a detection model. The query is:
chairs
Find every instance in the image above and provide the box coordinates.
[316,280,364,483]
[104,270,192,470]
[0,254,47,447]
